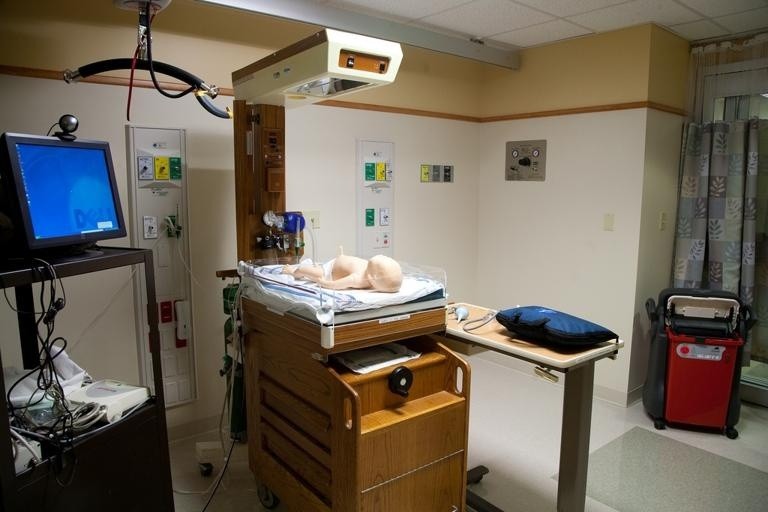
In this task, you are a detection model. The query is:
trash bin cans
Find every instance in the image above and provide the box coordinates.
[642,287,758,439]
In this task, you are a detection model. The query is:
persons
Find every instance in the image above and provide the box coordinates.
[279,246,404,294]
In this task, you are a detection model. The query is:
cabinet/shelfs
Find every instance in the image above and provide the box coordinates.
[240,295,472,512]
[0,245,177,512]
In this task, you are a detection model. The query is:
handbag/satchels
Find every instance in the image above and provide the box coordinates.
[496,306,618,351]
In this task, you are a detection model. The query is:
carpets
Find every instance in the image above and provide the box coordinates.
[551,425,768,511]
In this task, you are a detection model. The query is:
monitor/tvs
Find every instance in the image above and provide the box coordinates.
[4,132,127,249]
